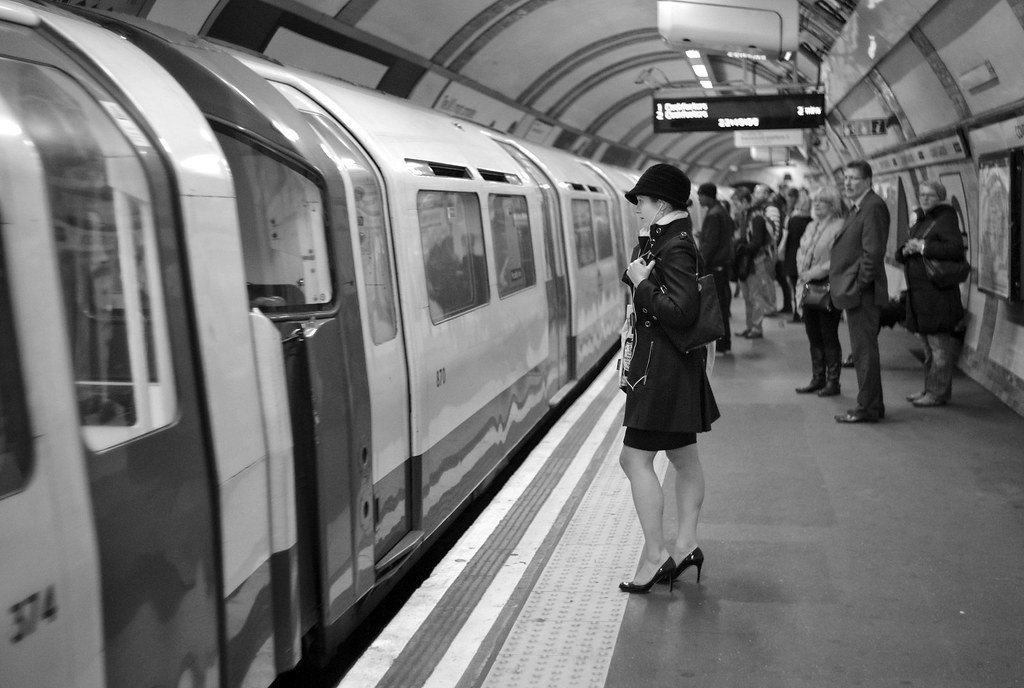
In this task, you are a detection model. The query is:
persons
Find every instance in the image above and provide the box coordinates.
[828,159,891,423]
[615,162,722,595]
[416,206,610,323]
[894,180,966,407]
[794,186,848,396]
[690,182,735,356]
[841,284,915,368]
[720,168,818,340]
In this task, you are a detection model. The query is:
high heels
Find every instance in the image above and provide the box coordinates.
[619,556,675,592]
[657,547,703,584]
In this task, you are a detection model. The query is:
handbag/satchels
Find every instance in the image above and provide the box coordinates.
[650,230,726,352]
[922,220,970,286]
[619,306,718,392]
[898,291,917,336]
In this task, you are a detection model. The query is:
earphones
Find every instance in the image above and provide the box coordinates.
[657,203,664,214]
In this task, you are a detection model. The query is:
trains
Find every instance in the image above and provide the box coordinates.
[0,1,735,687]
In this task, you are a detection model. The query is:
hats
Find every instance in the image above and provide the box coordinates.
[626,164,692,208]
[801,284,834,312]
[698,184,716,197]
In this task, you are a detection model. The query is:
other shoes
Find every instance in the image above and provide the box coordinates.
[906,392,924,400]
[835,414,880,423]
[844,357,854,368]
[746,331,763,338]
[736,330,750,335]
[913,394,945,406]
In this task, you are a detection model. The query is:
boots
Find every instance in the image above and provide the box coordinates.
[796,348,826,393]
[818,350,842,397]
